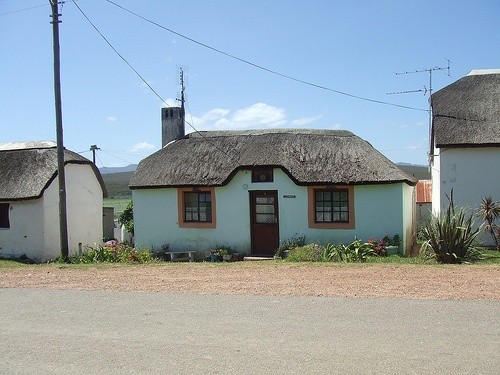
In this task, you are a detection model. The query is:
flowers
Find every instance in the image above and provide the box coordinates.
[208,244,236,256]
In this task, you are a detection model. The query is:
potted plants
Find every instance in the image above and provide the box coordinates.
[275,233,305,260]
[381,232,401,258]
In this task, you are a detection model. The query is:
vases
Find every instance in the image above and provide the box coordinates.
[209,253,239,262]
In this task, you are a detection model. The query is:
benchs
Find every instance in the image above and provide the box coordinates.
[164,250,196,263]
